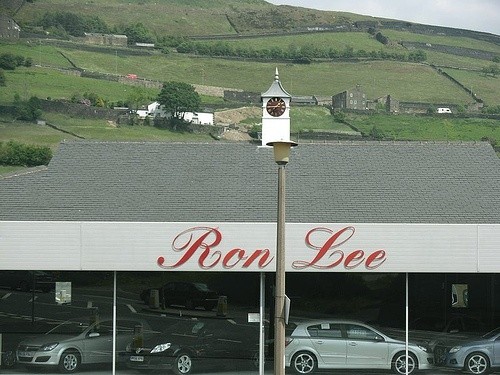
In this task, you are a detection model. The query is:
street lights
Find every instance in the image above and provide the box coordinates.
[266,139,298,374]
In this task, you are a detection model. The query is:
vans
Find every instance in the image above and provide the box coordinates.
[437,108,452,114]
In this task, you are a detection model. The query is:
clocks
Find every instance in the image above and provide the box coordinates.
[266,97,287,117]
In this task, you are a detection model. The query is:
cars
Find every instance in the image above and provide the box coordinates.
[284,319,437,375]
[14,316,154,374]
[125,317,265,375]
[425,322,500,375]
